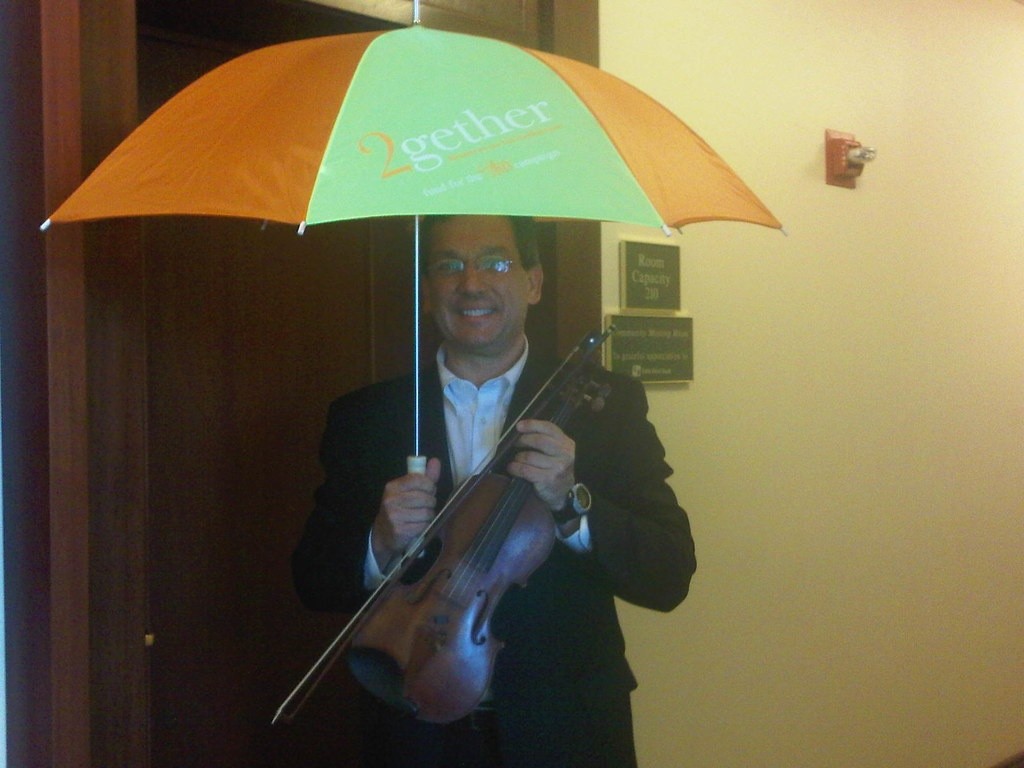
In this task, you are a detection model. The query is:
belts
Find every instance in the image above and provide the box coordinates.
[442,707,497,731]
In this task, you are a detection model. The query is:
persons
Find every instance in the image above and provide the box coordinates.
[289,214,696,768]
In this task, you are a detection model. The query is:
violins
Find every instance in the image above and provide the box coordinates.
[341,322,624,727]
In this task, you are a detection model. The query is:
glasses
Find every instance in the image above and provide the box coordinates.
[425,256,522,281]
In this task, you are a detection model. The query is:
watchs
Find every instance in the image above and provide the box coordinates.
[552,483,592,523]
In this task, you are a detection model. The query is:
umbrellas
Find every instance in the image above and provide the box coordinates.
[38,0,780,557]
